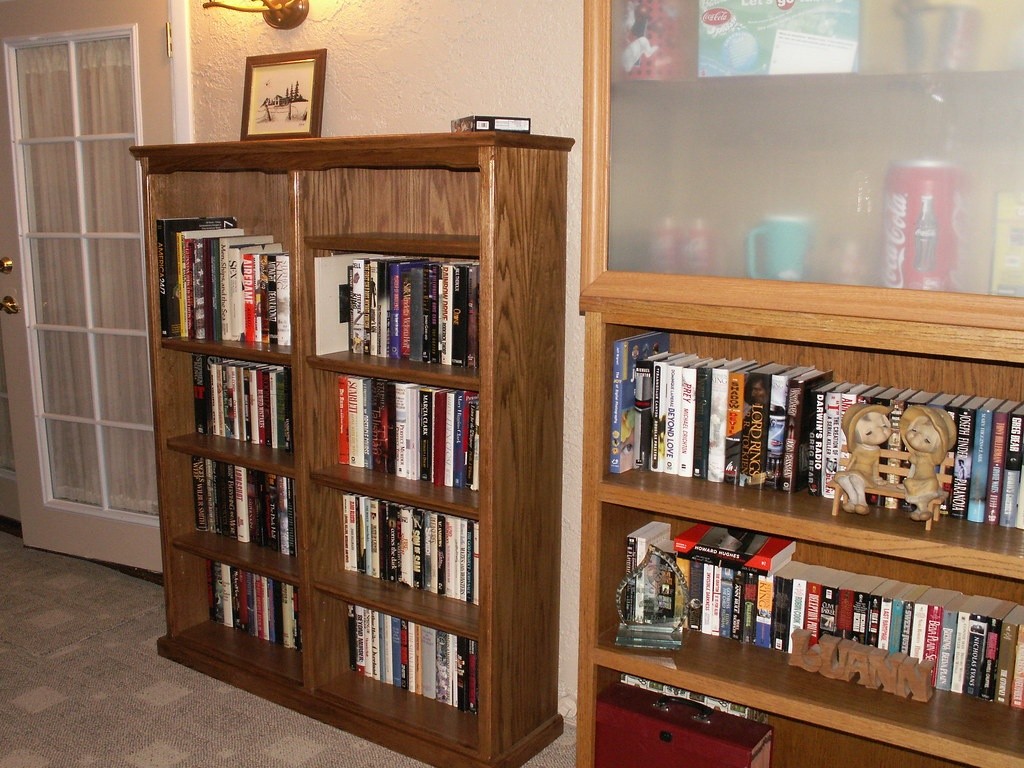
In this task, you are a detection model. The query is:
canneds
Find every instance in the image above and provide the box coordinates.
[877,160,967,293]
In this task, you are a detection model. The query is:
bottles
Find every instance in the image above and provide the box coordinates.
[914,195,938,270]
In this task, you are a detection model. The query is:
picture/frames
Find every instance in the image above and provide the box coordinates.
[240,48,328,141]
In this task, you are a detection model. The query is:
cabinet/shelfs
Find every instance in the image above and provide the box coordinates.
[575,296,1024,768]
[579,0,1024,332]
[128,131,575,768]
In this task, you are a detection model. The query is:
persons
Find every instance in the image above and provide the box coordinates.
[834,403,893,515]
[899,405,957,521]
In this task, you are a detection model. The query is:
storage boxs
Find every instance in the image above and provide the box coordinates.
[450,116,531,134]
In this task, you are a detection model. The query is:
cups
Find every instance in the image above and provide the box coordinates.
[747,213,810,280]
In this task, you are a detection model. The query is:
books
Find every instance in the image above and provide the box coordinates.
[348,604,479,714]
[314,253,479,368]
[611,331,1024,529]
[342,493,480,606]
[156,215,291,346]
[192,456,297,557]
[612,522,1024,711]
[337,375,480,491]
[206,560,302,651]
[191,354,293,452]
